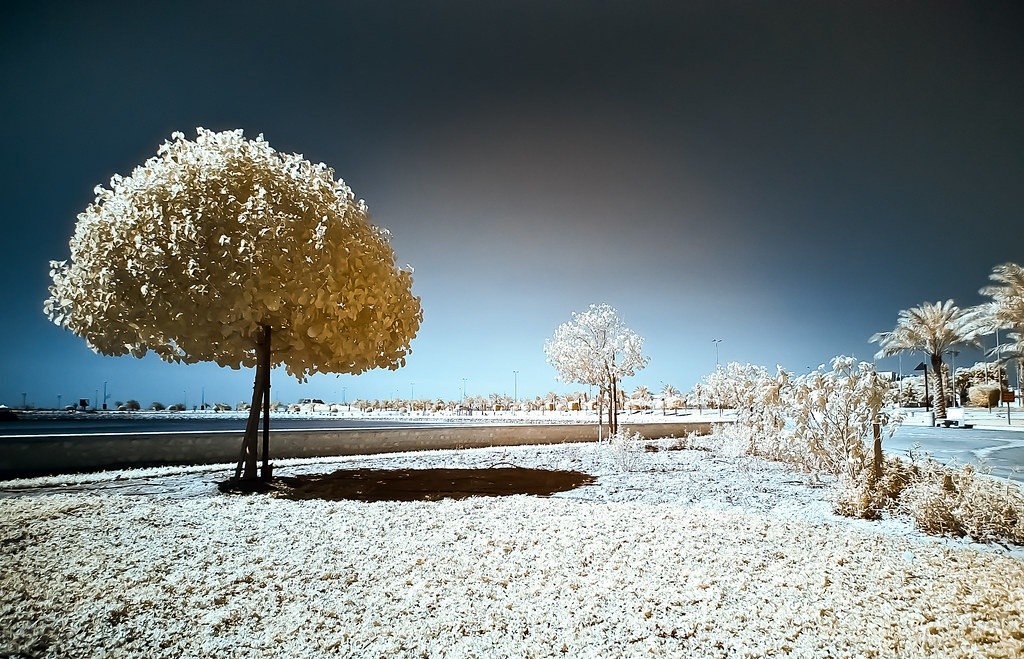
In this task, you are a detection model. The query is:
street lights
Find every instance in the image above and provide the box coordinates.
[512,370,520,402]
[410,383,414,399]
[946,350,960,408]
[463,378,468,395]
[711,338,723,363]
